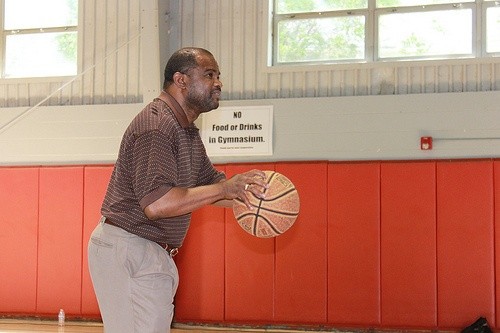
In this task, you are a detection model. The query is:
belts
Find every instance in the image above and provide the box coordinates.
[101,217,179,259]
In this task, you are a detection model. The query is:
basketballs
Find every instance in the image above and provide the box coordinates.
[233,171,300,238]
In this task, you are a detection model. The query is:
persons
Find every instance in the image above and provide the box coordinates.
[87,47,270,333]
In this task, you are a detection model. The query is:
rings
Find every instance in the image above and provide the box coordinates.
[244,184,249,191]
